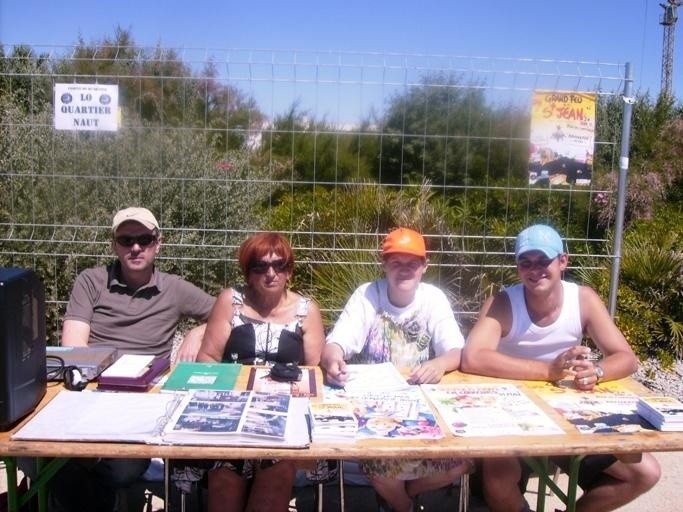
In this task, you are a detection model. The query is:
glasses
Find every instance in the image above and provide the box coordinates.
[113,234,159,246]
[251,259,288,274]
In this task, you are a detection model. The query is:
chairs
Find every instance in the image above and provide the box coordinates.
[140,328,178,511]
[331,330,465,510]
[182,329,328,510]
[468,330,559,511]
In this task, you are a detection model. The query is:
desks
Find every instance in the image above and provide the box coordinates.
[0,362,683,512]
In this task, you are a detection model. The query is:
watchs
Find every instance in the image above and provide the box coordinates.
[593,362,603,384]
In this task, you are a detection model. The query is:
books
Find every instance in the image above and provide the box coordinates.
[9,353,320,449]
[636,396,683,431]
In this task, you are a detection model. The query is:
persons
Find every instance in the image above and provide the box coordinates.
[40,205,217,510]
[318,225,476,511]
[196,232,332,511]
[458,223,662,511]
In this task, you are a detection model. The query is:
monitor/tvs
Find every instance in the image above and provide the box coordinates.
[0,268,47,428]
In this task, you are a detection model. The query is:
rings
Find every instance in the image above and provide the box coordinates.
[583,377,588,385]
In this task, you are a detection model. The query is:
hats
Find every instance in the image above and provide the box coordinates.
[515,224,563,264]
[383,228,426,259]
[112,207,160,231]
